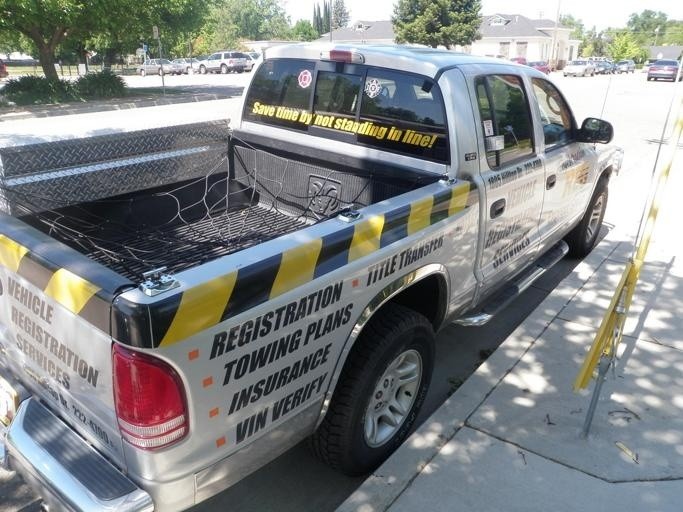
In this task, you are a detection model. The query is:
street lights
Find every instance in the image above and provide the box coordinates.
[653,25,661,47]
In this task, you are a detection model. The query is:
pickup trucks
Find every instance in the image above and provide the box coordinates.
[0,45,622,511]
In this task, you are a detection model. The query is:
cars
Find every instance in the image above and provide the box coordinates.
[0,60,8,79]
[508,56,679,83]
[136,51,260,77]
